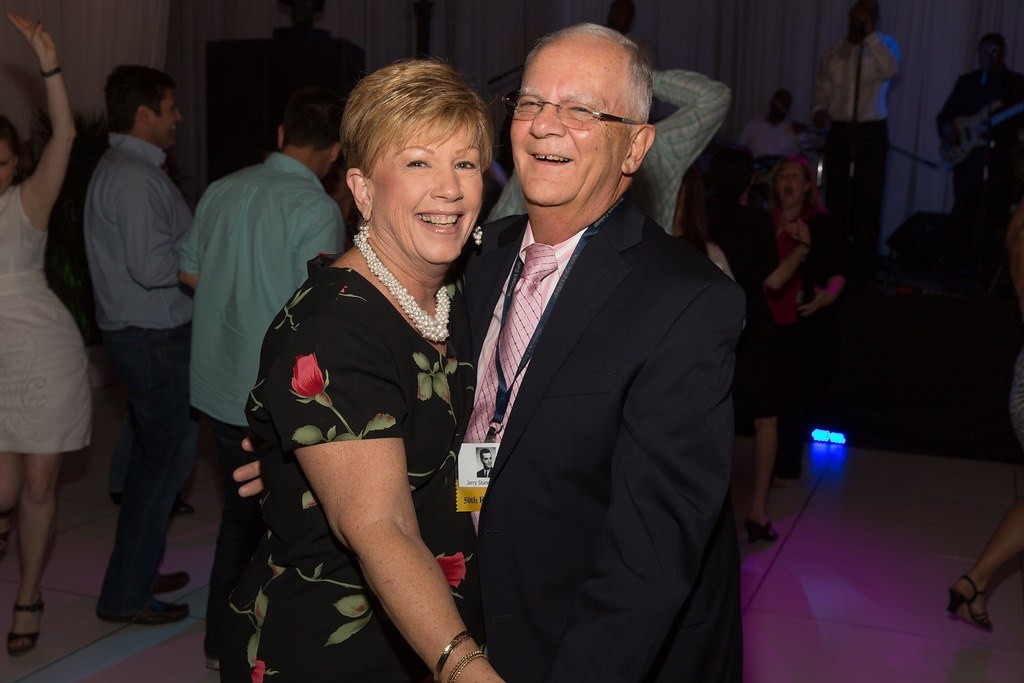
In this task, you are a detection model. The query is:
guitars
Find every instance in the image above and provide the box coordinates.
[939,100,1024,167]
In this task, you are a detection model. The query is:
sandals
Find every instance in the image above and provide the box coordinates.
[0,508,14,553]
[6,591,44,657]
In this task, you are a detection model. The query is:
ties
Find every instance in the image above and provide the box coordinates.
[463,244,555,539]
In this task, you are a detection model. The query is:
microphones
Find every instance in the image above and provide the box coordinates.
[859,16,868,22]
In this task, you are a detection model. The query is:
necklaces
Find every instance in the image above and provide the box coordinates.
[353,233,450,343]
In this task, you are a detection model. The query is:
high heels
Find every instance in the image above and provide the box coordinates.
[947,575,992,629]
[743,517,778,543]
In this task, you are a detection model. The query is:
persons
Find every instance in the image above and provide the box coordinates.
[224,59,506,683]
[0,12,93,655]
[811,0,901,255]
[740,88,811,158]
[84,64,201,627]
[233,23,747,683]
[703,145,847,541]
[180,86,346,669]
[607,0,636,35]
[477,448,493,478]
[485,68,731,236]
[936,32,1024,207]
[946,198,1024,631]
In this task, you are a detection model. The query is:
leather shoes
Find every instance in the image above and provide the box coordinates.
[97,594,190,625]
[146,571,189,594]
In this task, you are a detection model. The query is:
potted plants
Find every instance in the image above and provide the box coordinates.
[16,107,180,389]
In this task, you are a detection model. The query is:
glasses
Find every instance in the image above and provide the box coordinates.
[502,91,636,130]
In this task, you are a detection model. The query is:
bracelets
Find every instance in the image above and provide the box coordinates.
[447,650,488,683]
[41,65,62,77]
[433,631,471,683]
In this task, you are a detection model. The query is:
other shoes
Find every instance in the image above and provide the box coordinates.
[173,500,195,515]
